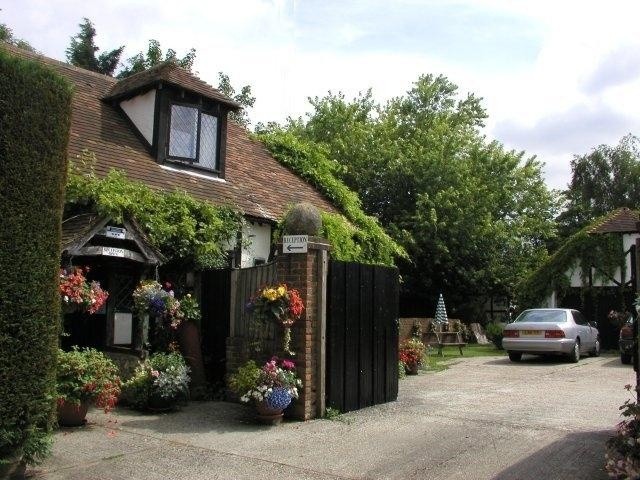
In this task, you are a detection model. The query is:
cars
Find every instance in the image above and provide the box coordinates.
[498,305,604,366]
[618,311,634,365]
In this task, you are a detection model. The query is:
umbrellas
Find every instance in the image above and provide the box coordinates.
[433,293,448,332]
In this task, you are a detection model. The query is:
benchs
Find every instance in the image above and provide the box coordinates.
[428,326,469,357]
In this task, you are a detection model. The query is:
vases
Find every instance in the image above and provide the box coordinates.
[55,405,90,429]
[257,401,285,425]
[147,392,178,412]
[280,324,296,355]
[405,363,419,376]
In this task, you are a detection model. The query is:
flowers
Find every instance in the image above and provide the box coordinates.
[60,263,109,317]
[400,338,432,377]
[246,282,305,326]
[55,346,121,414]
[229,357,305,415]
[132,277,202,329]
[120,341,193,398]
